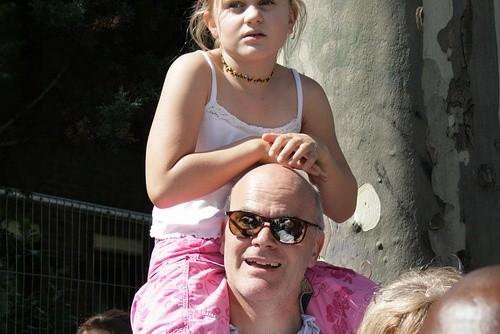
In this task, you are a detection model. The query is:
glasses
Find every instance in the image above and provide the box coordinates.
[224,210,322,245]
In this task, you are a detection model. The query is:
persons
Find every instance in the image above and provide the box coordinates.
[130,0,382,334]
[76,309,133,334]
[357,254,500,334]
[219,163,326,334]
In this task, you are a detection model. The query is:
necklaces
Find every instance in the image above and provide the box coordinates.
[220,53,274,83]
